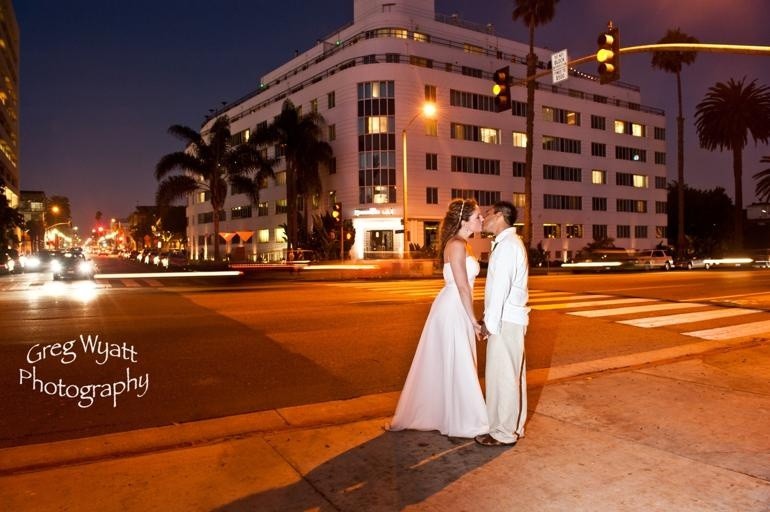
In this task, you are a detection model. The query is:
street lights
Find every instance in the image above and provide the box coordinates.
[398,98,439,253]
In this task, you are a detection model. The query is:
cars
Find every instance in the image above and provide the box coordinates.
[99,248,109,257]
[0,248,97,281]
[559,245,770,278]
[117,248,192,274]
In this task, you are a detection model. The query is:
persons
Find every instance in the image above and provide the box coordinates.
[476,200,531,446]
[392,198,490,439]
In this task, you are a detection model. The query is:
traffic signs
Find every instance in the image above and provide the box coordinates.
[551,48,568,85]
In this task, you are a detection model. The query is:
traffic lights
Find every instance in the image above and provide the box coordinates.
[330,201,344,225]
[490,64,515,114]
[598,28,623,87]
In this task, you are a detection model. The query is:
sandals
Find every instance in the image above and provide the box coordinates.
[474,434,516,446]
